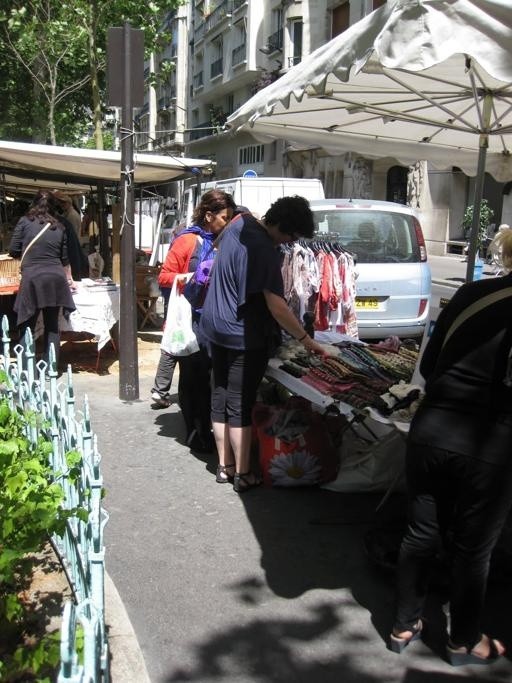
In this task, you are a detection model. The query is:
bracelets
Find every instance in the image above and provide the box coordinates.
[298,332,308,343]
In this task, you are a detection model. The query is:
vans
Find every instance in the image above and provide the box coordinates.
[309,198,431,339]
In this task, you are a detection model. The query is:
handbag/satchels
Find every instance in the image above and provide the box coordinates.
[183,212,251,309]
[252,402,338,488]
[326,427,407,492]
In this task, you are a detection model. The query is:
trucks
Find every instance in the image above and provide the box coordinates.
[180,177,326,220]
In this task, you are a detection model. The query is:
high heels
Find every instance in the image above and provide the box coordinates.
[446,635,502,665]
[390,616,432,654]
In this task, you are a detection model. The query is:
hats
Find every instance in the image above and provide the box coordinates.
[49,189,65,200]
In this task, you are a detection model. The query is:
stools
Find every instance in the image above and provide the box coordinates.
[137,296,159,330]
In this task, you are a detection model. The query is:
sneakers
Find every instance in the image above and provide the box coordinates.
[151,392,171,407]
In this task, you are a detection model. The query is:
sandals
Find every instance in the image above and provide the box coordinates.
[235,471,264,492]
[215,463,236,483]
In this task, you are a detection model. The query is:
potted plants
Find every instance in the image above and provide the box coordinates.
[461,198,495,281]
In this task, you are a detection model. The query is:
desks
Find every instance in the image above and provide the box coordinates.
[264,331,426,514]
[32,280,121,373]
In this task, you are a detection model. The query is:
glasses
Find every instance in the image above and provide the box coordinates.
[288,231,298,242]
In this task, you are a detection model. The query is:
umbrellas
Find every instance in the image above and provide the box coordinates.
[218,5,512,285]
[0,133,216,200]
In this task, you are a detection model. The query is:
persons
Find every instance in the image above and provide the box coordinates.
[387,228,512,642]
[150,207,250,407]
[199,196,325,493]
[157,191,237,452]
[7,190,77,379]
